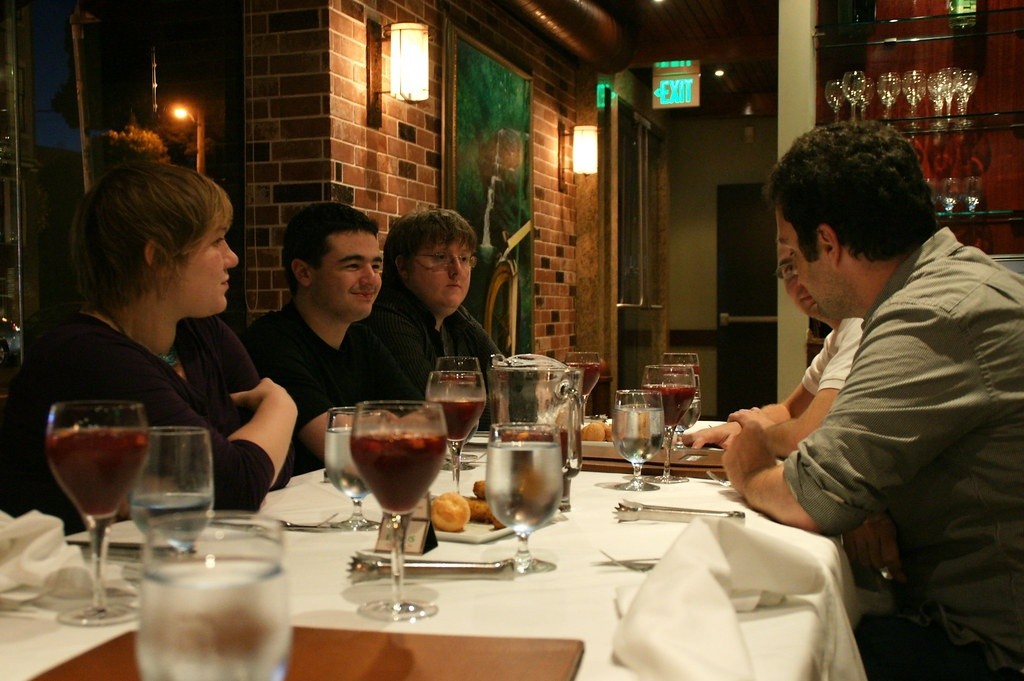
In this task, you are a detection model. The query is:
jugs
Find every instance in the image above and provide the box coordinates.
[485,352,585,513]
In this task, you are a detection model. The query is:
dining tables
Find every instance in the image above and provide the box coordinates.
[0,420,868,681]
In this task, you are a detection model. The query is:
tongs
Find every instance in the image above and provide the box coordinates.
[343,551,516,586]
[611,497,745,526]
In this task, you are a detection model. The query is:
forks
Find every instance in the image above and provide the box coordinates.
[598,548,655,572]
[707,471,731,489]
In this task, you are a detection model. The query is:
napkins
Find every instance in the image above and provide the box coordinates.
[610,515,825,680]
[0,509,84,610]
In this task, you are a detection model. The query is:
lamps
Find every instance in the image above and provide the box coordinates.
[365,18,430,127]
[557,120,598,193]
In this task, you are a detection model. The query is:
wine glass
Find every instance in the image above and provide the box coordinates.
[350,398,438,624]
[426,370,485,500]
[46,398,139,628]
[433,353,485,462]
[484,420,565,576]
[562,351,600,424]
[129,424,214,556]
[323,407,381,533]
[640,352,702,484]
[824,67,978,131]
[612,390,664,492]
[922,176,982,221]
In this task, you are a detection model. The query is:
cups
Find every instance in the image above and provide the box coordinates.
[135,510,293,680]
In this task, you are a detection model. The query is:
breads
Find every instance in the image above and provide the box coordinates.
[431,492,471,531]
[579,422,614,442]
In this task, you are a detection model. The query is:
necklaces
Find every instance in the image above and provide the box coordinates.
[96,305,178,367]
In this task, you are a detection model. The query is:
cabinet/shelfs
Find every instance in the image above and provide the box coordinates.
[812,7,1024,226]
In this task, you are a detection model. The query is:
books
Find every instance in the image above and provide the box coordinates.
[26,625,585,681]
[580,444,727,481]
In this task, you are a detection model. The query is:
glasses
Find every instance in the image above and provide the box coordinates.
[774,262,798,281]
[414,252,477,271]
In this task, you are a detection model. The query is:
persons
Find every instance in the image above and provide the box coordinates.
[362,201,538,431]
[0,161,299,536]
[239,202,426,478]
[665,120,1024,681]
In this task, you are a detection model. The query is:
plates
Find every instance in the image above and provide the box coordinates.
[433,509,561,544]
